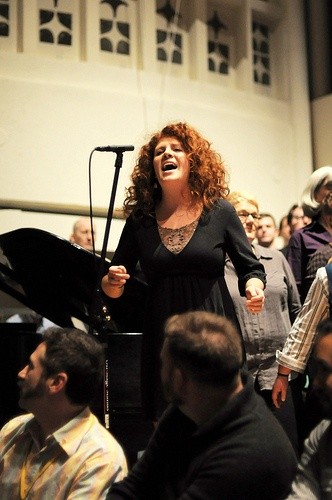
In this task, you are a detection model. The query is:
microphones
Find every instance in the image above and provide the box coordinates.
[95,145,134,152]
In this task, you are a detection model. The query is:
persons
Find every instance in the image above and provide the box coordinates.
[0,328,128,500]
[256,203,310,254]
[286,318,331,500]
[224,191,302,392]
[69,218,96,251]
[99,123,267,423]
[271,256,331,409]
[307,243,331,296]
[105,312,297,500]
[288,167,332,297]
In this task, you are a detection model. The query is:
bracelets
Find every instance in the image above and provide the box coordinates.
[277,371,289,377]
